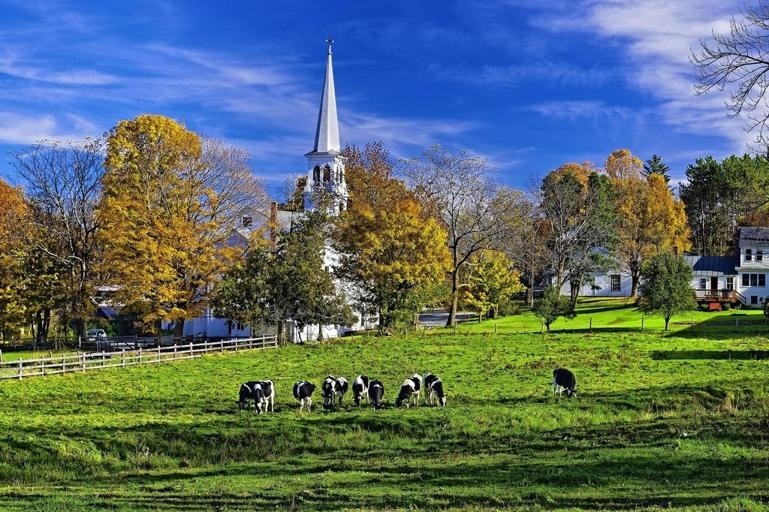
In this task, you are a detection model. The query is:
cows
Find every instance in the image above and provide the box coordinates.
[293,380,316,413]
[235,380,275,414]
[552,369,577,398]
[320,375,348,415]
[424,373,448,407]
[369,378,385,412]
[352,375,369,407]
[395,373,422,409]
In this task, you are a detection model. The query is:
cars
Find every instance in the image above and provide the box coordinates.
[87,328,107,341]
